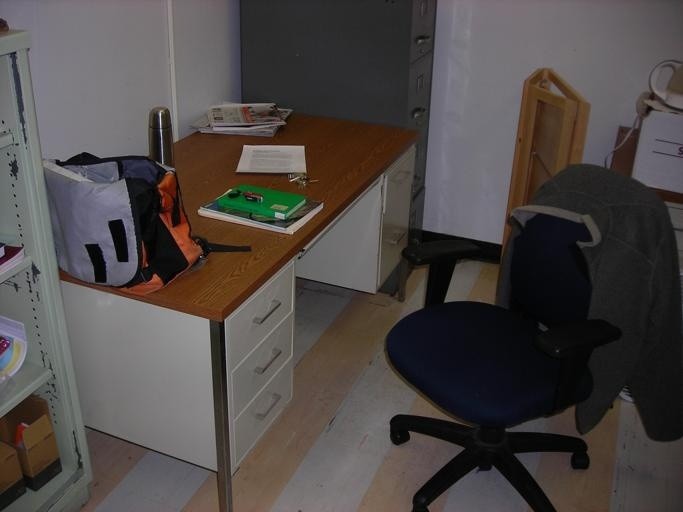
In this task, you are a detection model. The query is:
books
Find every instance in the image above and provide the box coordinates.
[0,240,28,383]
[42,158,94,184]
[189,106,294,138]
[197,182,327,236]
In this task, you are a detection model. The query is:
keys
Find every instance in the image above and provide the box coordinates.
[287,172,320,190]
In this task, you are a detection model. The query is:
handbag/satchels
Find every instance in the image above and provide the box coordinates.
[42,152,202,297]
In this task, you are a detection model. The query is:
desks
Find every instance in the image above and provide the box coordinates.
[50,109,415,510]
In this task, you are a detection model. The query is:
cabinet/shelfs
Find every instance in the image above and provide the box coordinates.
[0,27,100,512]
[238,0,442,297]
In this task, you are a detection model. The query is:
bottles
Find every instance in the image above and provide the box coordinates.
[148,106,174,170]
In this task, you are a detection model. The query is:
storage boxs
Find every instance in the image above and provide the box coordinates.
[0,396,63,511]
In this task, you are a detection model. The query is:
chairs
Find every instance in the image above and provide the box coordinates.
[386,164,675,512]
